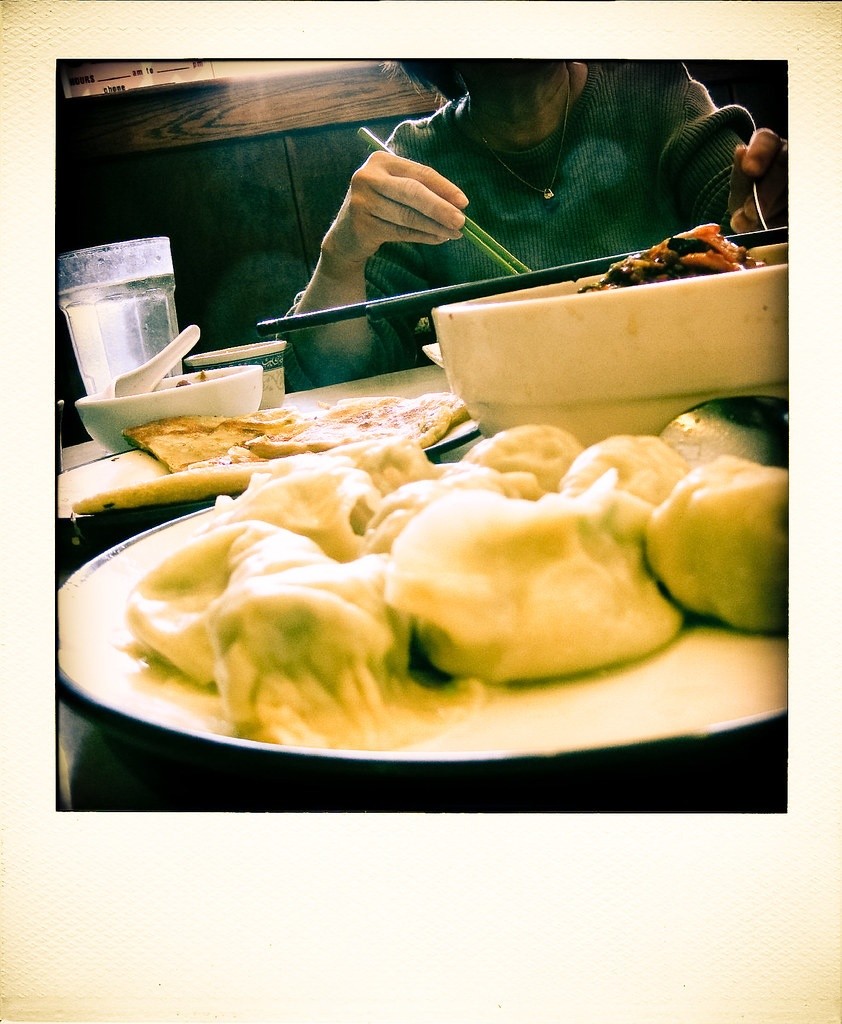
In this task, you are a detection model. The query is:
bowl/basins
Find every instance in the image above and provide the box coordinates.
[74,365,266,456]
[431,240,789,449]
[182,339,285,412]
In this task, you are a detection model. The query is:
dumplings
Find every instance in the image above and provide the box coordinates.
[116,424,789,755]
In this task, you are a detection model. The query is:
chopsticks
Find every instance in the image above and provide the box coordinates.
[362,128,536,277]
[257,219,789,340]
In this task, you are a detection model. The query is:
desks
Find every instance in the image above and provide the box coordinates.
[61,362,788,813]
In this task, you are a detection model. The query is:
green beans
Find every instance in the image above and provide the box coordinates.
[666,236,711,254]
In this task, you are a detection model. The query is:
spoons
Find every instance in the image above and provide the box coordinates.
[658,394,789,473]
[114,324,201,397]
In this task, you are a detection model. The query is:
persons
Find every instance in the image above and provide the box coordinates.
[271,58,788,391]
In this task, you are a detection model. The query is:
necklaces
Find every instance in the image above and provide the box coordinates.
[468,72,570,200]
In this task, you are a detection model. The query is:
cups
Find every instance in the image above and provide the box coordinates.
[56,236,185,396]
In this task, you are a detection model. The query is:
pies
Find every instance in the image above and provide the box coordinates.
[246,390,469,451]
[119,402,332,473]
[73,446,376,515]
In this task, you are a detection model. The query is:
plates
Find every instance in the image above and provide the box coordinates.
[56,398,481,548]
[57,481,788,764]
[421,343,444,370]
